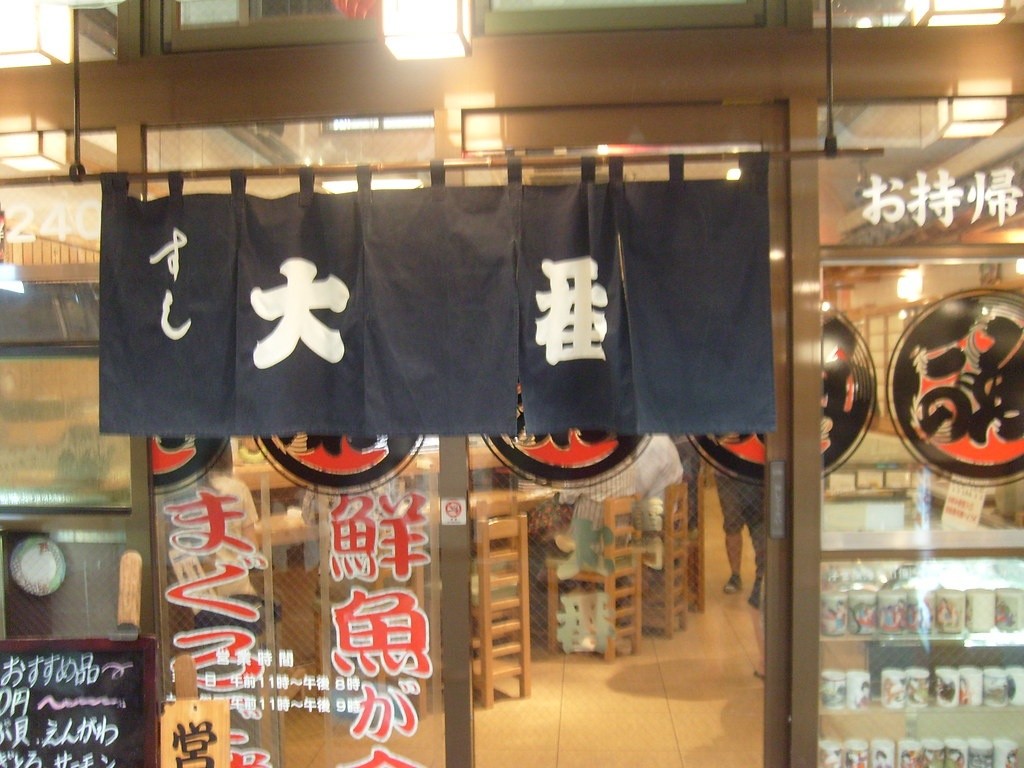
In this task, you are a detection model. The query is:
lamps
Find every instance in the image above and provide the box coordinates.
[0,0,72,68]
[935,97,1012,140]
[376,0,474,64]
[910,0,1017,27]
[0,131,72,172]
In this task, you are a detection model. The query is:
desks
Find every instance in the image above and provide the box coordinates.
[252,489,540,549]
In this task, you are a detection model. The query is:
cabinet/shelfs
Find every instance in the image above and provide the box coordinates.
[819,527,1024,768]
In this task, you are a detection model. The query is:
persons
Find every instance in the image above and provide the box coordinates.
[528,430,684,604]
[163,430,262,634]
[714,464,769,613]
[301,436,402,716]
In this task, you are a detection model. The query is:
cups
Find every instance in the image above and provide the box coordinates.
[869,737,894,768]
[992,740,1018,768]
[983,669,1007,706]
[818,740,841,768]
[958,667,983,705]
[819,566,1024,636]
[1006,667,1023,705]
[921,739,945,768]
[881,670,904,708]
[845,670,870,709]
[945,738,968,768]
[897,740,922,768]
[968,738,992,768]
[934,668,958,707]
[819,669,845,710]
[843,740,868,768]
[904,669,930,707]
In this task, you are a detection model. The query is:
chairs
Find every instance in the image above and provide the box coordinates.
[544,492,643,665]
[168,543,285,673]
[424,509,532,710]
[476,495,518,577]
[615,481,690,640]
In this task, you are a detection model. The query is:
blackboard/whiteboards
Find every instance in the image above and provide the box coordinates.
[0,636,161,768]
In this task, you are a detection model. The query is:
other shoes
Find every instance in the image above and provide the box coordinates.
[748,587,761,610]
[724,575,742,594]
